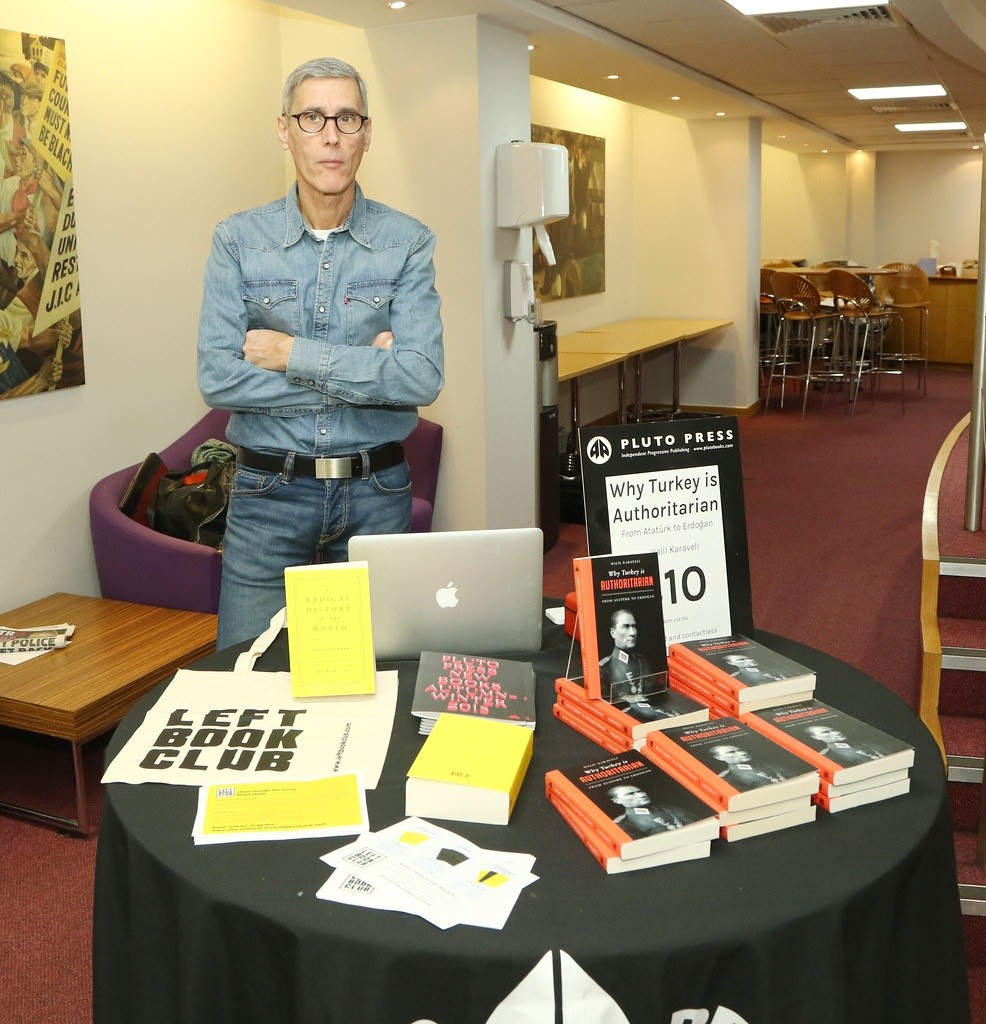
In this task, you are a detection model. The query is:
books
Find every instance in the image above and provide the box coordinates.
[544,634,916,882]
[572,552,670,700]
[411,650,537,735]
[283,560,378,698]
[405,714,533,826]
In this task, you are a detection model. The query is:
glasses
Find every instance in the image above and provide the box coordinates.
[281,109,371,134]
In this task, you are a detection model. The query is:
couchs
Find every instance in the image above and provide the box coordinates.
[89,392,443,614]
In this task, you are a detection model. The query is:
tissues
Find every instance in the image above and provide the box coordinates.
[496,142,569,265]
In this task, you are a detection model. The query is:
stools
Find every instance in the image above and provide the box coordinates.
[759,262,933,418]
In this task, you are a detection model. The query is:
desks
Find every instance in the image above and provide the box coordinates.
[554,313,735,479]
[91,595,971,1024]
[766,266,900,390]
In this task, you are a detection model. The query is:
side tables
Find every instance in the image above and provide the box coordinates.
[0,590,218,838]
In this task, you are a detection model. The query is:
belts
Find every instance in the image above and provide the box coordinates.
[234,452,408,482]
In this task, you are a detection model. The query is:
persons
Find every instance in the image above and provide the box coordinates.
[197,56,447,653]
[598,610,664,696]
[724,653,792,687]
[606,783,694,839]
[805,726,890,770]
[709,740,794,792]
